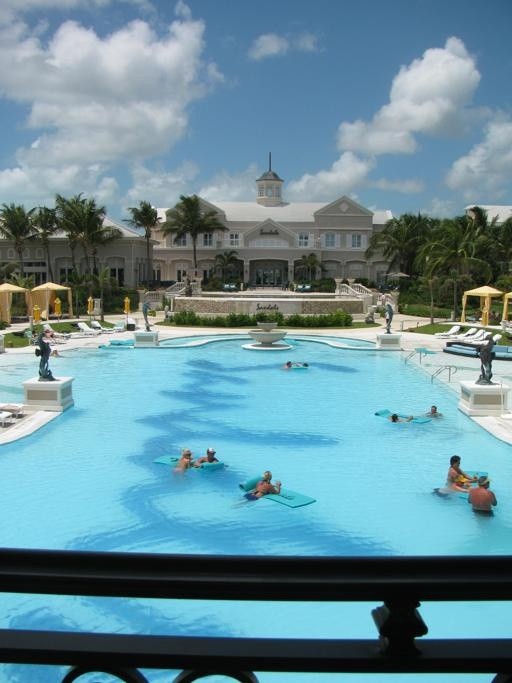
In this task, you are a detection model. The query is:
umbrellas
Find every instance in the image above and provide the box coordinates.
[482,312,487,341]
[123,297,130,324]
[87,296,94,328]
[54,297,62,323]
[32,305,41,324]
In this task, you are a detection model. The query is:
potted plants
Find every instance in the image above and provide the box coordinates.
[254,310,284,331]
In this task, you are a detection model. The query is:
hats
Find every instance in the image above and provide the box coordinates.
[478,477,492,485]
[207,447,216,454]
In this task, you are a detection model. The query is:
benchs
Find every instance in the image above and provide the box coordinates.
[441,342,511,359]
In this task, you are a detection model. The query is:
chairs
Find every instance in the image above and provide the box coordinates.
[433,325,502,346]
[24,324,71,346]
[76,320,114,336]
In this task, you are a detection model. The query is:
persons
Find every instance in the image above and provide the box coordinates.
[385,311,389,324]
[388,405,443,423]
[243,471,281,502]
[32,329,65,341]
[472,310,501,321]
[283,361,308,369]
[173,447,219,474]
[433,455,497,516]
